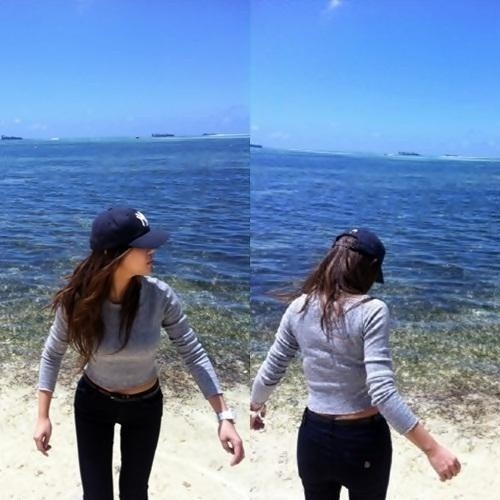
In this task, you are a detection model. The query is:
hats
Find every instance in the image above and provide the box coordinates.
[89,206,171,250]
[332,227,386,284]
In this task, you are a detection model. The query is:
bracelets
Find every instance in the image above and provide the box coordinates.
[216,408,238,425]
[248,403,269,422]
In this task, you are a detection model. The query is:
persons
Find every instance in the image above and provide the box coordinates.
[247,226,460,499]
[25,199,247,499]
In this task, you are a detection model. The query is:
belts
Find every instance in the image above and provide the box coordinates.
[307,408,381,426]
[82,372,160,402]
